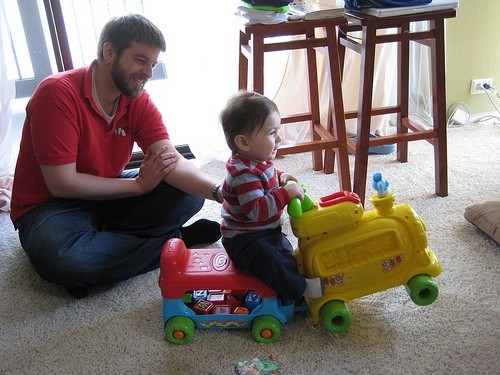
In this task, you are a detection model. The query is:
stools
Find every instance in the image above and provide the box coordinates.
[239,18,351,192]
[324,9,457,211]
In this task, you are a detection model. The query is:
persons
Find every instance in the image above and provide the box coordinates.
[8,13,222,302]
[220,89,326,299]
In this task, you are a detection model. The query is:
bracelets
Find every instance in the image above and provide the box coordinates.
[212,183,223,203]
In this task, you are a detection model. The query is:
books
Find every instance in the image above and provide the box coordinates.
[350,0,460,20]
[287,1,345,21]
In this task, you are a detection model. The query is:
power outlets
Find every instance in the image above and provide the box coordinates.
[470,78,492,95]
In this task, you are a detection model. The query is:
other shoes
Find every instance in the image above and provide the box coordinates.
[348,133,394,154]
